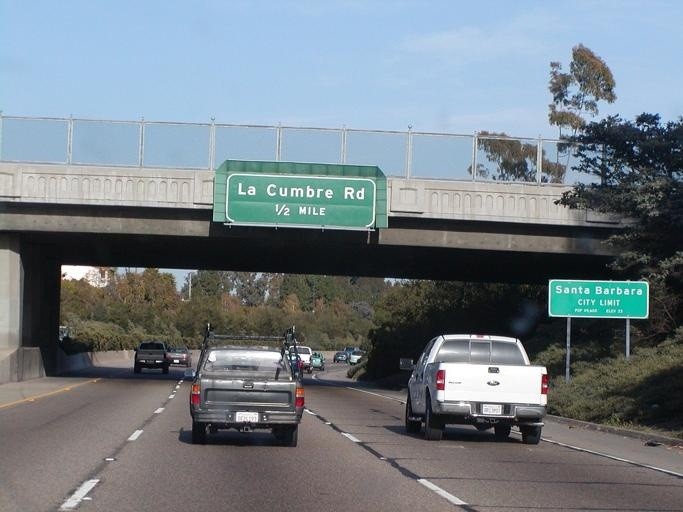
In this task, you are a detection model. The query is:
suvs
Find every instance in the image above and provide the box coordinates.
[131,339,172,376]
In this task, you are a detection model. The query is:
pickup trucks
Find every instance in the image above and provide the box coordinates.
[189,322,305,448]
[397,331,549,446]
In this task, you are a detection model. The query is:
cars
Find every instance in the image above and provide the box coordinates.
[167,345,193,368]
[286,339,367,378]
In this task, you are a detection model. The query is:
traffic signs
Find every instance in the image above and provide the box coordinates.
[549,280,649,319]
[224,172,378,232]
